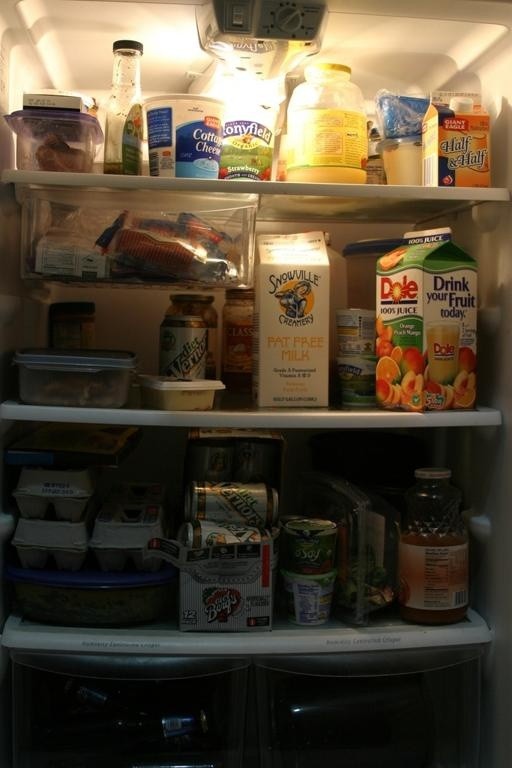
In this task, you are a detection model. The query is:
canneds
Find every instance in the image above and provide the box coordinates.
[159,289,260,407]
[176,442,280,550]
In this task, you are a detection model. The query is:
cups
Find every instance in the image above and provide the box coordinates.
[424,324,461,384]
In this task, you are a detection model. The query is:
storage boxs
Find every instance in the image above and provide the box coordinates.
[6,652,250,765]
[254,648,482,765]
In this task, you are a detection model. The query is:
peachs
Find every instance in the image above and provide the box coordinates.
[375,339,478,412]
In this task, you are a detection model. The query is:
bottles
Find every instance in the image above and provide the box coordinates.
[46,299,102,354]
[341,237,409,313]
[283,62,369,186]
[160,287,264,392]
[101,40,146,176]
[396,464,471,626]
